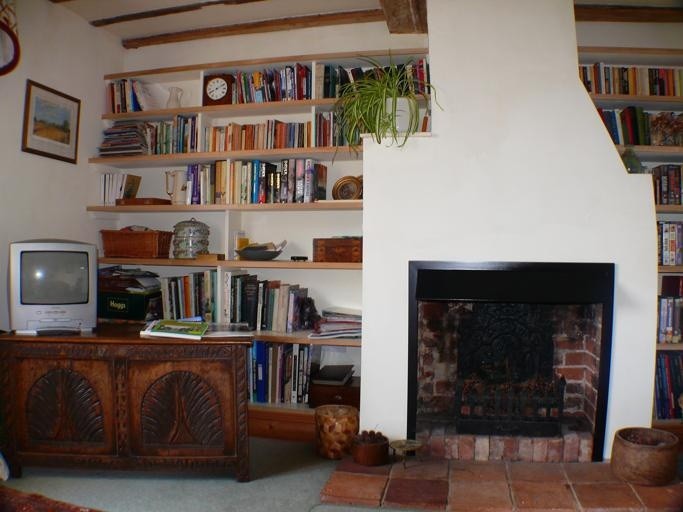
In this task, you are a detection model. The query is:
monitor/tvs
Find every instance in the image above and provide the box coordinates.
[10,243,98,336]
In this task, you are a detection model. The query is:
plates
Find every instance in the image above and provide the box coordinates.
[234,249,281,259]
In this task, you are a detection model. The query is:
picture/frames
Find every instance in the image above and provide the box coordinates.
[20,78,81,166]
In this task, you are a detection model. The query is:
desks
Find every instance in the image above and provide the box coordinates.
[1,318,253,483]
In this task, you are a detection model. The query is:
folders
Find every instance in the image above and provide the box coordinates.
[322,306,362,322]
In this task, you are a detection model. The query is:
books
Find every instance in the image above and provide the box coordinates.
[316,306,361,339]
[104,76,163,113]
[655,352,681,420]
[596,104,682,144]
[657,274,683,344]
[247,340,321,405]
[313,363,355,386]
[148,114,198,155]
[222,268,309,334]
[138,317,210,342]
[96,119,149,155]
[642,164,682,205]
[202,118,312,151]
[230,158,329,204]
[183,160,227,204]
[231,61,311,103]
[314,107,361,146]
[578,66,683,96]
[159,269,218,322]
[656,219,683,265]
[315,58,429,98]
[98,171,143,205]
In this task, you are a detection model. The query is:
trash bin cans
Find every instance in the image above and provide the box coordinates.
[315,404,358,460]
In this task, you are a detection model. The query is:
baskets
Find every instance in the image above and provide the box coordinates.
[99,228,177,259]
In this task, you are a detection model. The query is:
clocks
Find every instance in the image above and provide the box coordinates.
[202,73,235,106]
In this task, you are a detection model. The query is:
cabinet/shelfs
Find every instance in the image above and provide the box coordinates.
[84,47,433,442]
[578,44,683,451]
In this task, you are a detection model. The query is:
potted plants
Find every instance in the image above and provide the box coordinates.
[331,47,444,165]
